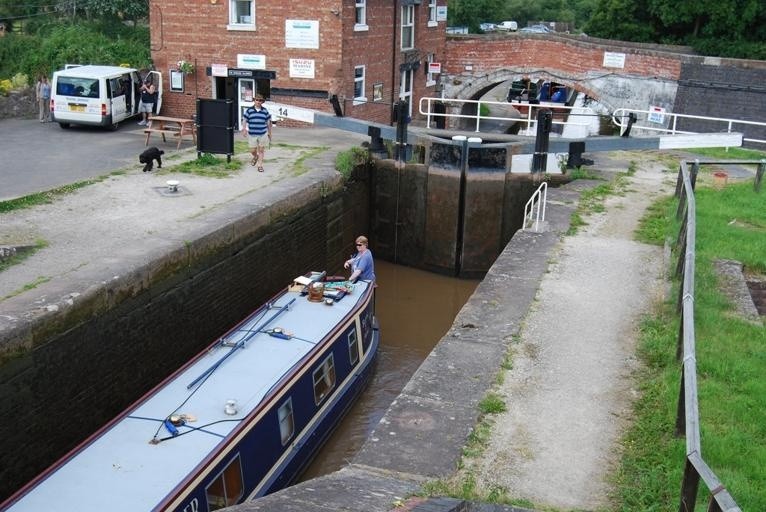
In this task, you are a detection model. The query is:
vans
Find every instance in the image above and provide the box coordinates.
[497,21,517,32]
[49,66,163,131]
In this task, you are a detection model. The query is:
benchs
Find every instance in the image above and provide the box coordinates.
[163,125,196,133]
[144,129,181,137]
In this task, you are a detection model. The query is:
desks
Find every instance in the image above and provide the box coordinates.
[146,116,195,150]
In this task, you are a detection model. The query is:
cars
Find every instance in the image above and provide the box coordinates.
[520,25,552,34]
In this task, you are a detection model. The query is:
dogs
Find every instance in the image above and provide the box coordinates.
[138,145,165,172]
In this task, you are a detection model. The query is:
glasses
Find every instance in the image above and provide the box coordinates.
[356,244,364,247]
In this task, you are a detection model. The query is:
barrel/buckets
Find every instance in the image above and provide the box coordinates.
[166,180,180,192]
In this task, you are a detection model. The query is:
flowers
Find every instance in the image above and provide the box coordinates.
[176,61,193,75]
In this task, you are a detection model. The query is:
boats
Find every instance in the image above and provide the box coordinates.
[507,77,574,122]
[0,271,379,512]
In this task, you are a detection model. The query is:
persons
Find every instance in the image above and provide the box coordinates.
[242,93,272,173]
[343,235,375,284]
[34,72,52,123]
[136,76,155,128]
[520,74,531,88]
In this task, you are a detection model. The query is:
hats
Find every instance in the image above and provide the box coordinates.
[144,79,150,85]
[252,94,265,103]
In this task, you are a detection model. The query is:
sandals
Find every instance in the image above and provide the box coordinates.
[251,155,264,173]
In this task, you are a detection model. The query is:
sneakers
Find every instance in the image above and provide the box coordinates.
[136,119,152,127]
[39,118,53,123]
